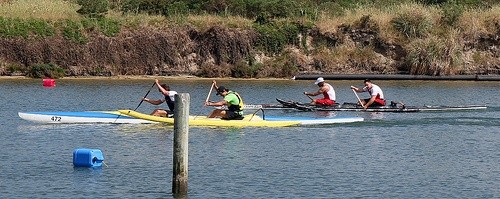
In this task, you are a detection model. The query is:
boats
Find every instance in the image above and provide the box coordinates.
[16,96,489,128]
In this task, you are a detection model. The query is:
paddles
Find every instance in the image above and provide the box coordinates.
[304,92,313,100]
[134,82,156,111]
[194,83,215,120]
[351,86,366,109]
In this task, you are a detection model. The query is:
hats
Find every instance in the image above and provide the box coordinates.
[159,83,169,92]
[315,78,324,85]
[216,86,226,95]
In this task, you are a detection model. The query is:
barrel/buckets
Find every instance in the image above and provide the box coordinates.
[72,149,104,168]
[42,79,56,87]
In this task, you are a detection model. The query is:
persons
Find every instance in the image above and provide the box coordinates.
[350,79,385,109]
[304,77,336,106]
[141,79,178,118]
[204,79,244,120]
